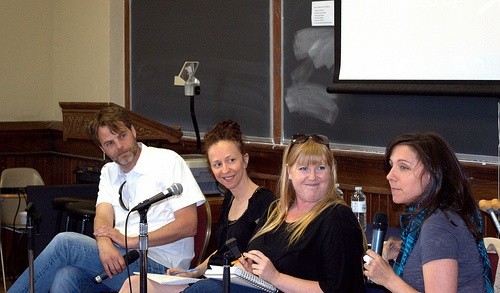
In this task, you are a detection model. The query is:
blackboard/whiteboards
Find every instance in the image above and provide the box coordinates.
[123,1,277,147]
[278,1,500,167]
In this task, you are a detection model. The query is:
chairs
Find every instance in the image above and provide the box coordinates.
[189,200,212,270]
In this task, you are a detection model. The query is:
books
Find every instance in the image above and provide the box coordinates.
[203,264,278,293]
[133,271,203,286]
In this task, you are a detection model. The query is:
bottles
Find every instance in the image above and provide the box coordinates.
[335,184,343,200]
[351,187,366,232]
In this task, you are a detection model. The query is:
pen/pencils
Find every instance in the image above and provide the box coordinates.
[169,267,201,275]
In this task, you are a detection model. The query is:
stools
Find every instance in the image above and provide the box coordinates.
[52,197,96,235]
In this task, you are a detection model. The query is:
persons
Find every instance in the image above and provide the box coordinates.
[6,104,207,293]
[119,120,278,293]
[363,133,495,293]
[179,134,367,293]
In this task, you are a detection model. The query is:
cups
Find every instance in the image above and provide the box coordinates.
[18,212,27,224]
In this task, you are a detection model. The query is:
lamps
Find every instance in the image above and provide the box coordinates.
[0,168,45,276]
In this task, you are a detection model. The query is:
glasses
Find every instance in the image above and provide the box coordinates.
[285,133,330,165]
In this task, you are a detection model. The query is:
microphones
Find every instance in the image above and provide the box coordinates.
[132,184,183,211]
[95,250,139,283]
[366,212,386,284]
